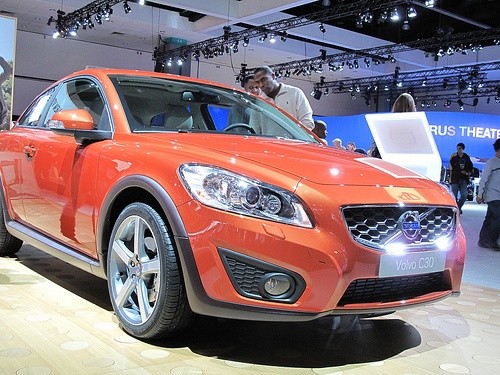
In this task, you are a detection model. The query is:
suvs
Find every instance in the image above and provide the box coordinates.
[0,65,467,343]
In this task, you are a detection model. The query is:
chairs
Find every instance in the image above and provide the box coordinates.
[161,104,193,128]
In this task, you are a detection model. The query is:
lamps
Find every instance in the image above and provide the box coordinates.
[47,1,131,38]
[150,0,500,109]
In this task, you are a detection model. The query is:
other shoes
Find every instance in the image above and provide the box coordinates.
[459,210,462,214]
[477,239,500,251]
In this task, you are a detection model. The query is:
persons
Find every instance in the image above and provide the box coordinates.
[248,65,315,138]
[312,120,328,139]
[449,143,474,215]
[332,138,346,149]
[228,76,261,134]
[476,138,500,251]
[347,142,356,151]
[367,93,416,159]
